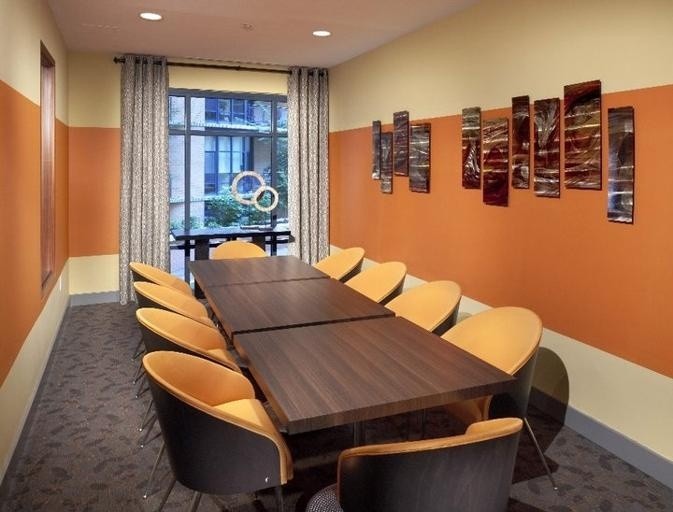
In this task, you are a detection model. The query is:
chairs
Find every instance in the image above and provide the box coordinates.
[127,259,193,361]
[211,239,267,260]
[382,278,465,336]
[342,259,409,305]
[131,280,220,385]
[137,350,297,512]
[127,306,246,451]
[313,244,365,281]
[435,303,556,492]
[332,416,525,511]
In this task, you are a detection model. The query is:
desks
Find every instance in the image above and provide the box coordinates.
[172,225,292,298]
[189,253,522,502]
